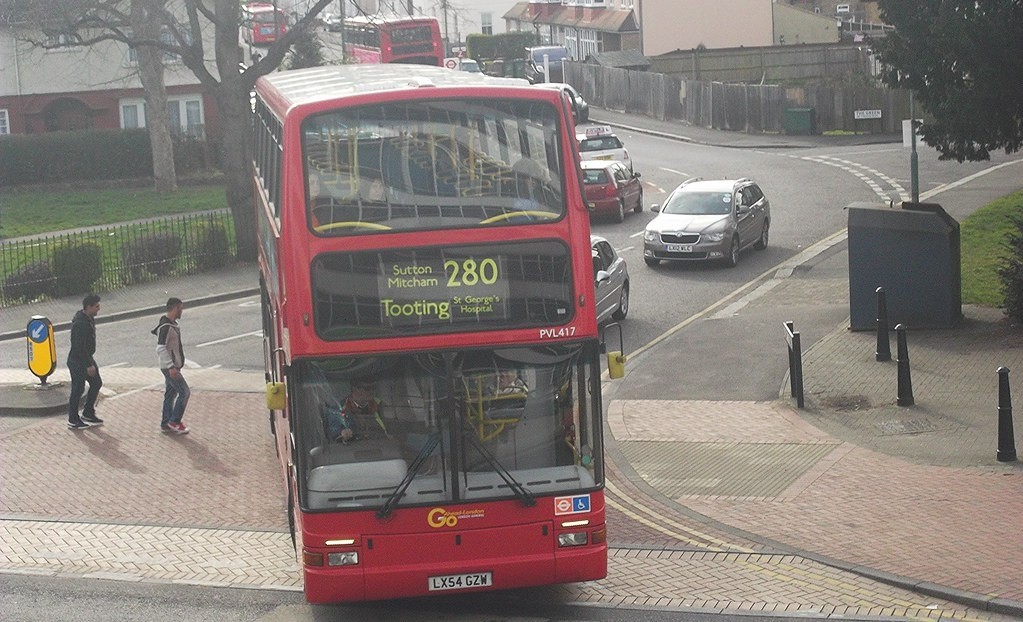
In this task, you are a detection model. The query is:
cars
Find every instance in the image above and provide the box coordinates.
[590,235,630,325]
[533,83,590,122]
[581,160,643,224]
[576,133,633,172]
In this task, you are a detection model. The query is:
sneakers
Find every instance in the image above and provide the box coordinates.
[68,418,90,429]
[168,421,189,433]
[81,415,103,425]
[160,425,177,433]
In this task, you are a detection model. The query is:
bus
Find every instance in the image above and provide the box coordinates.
[341,13,444,67]
[240,3,289,46]
[252,62,625,604]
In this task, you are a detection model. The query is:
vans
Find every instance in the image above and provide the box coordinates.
[442,58,483,75]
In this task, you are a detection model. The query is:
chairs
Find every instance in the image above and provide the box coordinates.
[597,173,609,184]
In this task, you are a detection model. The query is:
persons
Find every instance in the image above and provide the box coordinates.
[329,375,385,442]
[252,50,261,64]
[151,297,190,433]
[486,370,522,394]
[309,169,389,233]
[66,294,103,429]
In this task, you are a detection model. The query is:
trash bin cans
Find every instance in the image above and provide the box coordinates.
[784,107,816,136]
[842,200,962,332]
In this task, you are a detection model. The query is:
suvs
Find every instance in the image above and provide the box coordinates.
[644,176,771,268]
[525,47,573,83]
